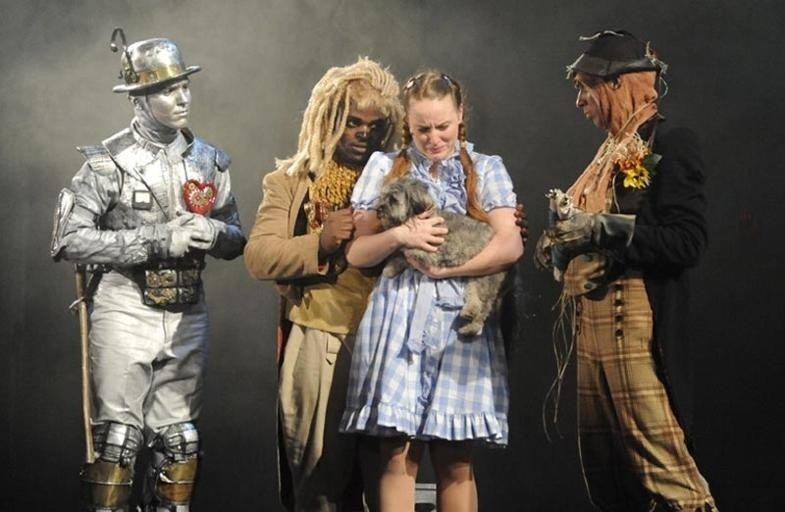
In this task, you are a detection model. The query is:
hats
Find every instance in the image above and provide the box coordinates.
[113,37,202,93]
[570,30,660,79]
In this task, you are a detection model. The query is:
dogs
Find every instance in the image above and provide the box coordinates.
[372,173,511,337]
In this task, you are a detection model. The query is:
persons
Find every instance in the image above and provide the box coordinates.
[531,27,723,511]
[243,56,532,511]
[341,69,525,512]
[51,36,247,512]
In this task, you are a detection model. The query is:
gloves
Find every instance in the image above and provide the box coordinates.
[532,212,638,274]
[164,208,217,259]
